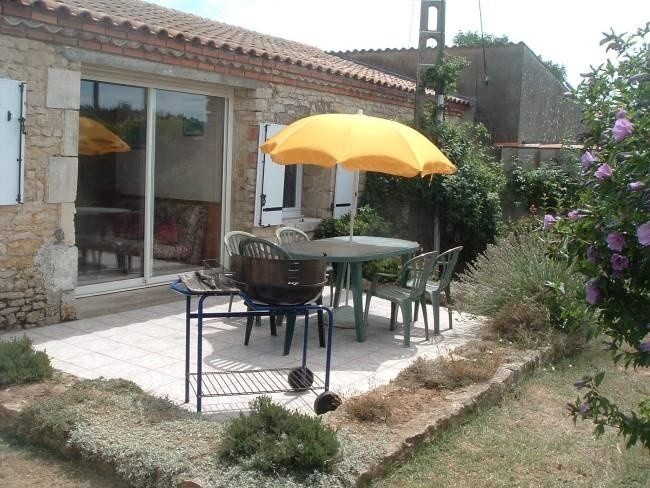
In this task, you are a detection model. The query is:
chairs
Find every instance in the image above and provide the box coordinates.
[222,226,464,356]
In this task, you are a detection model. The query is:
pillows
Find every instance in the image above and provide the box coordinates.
[112,209,179,246]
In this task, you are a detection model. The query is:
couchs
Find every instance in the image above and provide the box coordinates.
[112,197,207,265]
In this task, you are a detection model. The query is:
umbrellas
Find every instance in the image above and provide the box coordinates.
[257,108,459,307]
[80,116,131,155]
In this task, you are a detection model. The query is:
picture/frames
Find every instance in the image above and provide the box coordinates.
[126,121,146,149]
[183,99,204,135]
